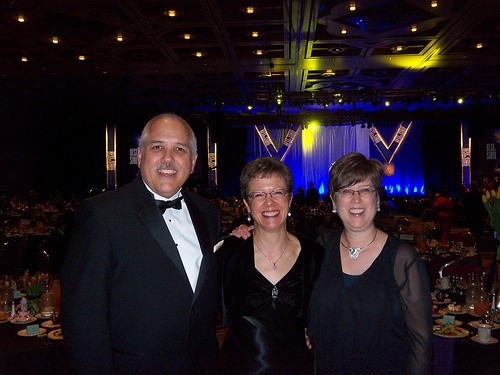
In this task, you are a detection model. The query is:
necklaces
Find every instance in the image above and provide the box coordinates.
[254,236,289,270]
[341,228,377,260]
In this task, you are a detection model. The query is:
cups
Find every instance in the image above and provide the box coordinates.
[436,292,445,302]
[478,324,493,342]
[436,277,449,289]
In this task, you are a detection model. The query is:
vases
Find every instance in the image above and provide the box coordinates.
[27,295,40,315]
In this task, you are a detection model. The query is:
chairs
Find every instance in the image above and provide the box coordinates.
[427,251,483,284]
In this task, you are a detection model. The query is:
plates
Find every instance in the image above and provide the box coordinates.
[432,301,500,343]
[10,311,63,340]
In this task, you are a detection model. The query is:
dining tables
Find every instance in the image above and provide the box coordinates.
[433,289,500,375]
[0,319,64,375]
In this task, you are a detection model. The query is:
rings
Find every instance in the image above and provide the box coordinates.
[236,225,243,230]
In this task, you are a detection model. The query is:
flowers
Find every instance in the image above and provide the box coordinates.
[17,269,49,312]
[482,186,500,260]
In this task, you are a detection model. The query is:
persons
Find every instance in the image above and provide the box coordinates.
[306,181,320,206]
[60,111,221,375]
[229,151,434,375]
[433,167,500,252]
[294,187,305,206]
[211,156,326,375]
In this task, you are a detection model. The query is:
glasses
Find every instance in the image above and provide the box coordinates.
[247,187,290,202]
[337,186,378,200]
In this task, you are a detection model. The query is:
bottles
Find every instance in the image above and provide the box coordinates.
[40,279,54,317]
[0,275,11,321]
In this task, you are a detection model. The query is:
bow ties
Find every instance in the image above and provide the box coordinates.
[155,195,184,214]
